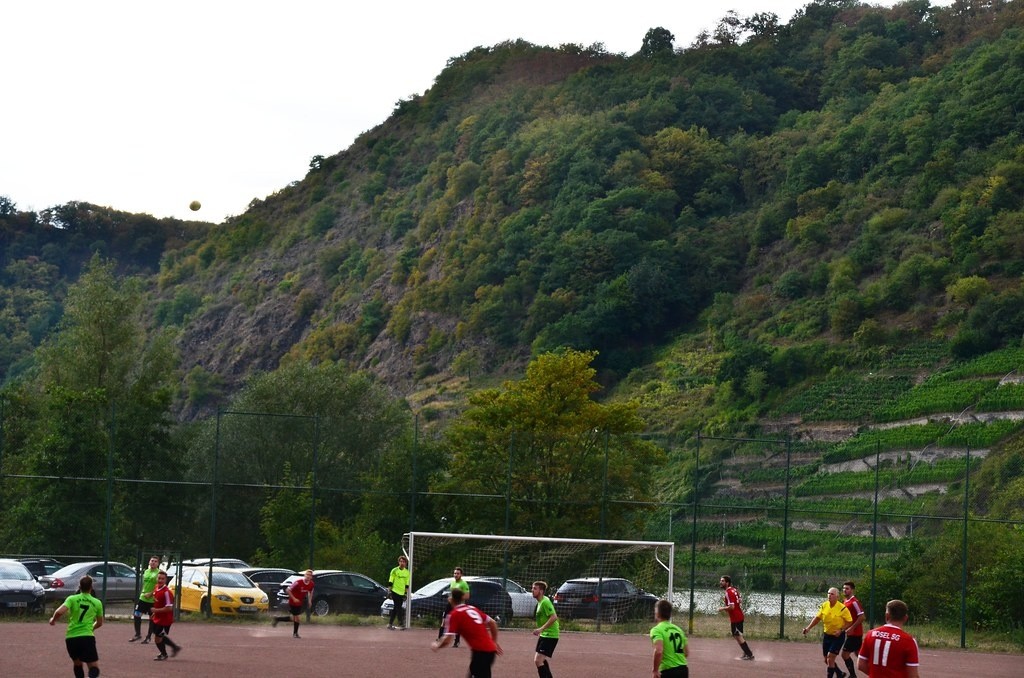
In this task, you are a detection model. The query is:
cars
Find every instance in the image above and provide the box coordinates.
[37,562,141,605]
[15,556,70,582]
[381,576,538,629]
[158,558,297,621]
[0,559,47,615]
[276,570,389,618]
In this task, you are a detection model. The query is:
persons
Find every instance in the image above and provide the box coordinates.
[841,582,864,678]
[272,569,315,638]
[649,600,690,678]
[129,556,163,644]
[432,588,504,678]
[531,581,559,678]
[858,600,919,678]
[150,571,181,660]
[718,575,754,660]
[436,567,470,647]
[803,587,853,678]
[50,576,103,678]
[387,556,410,630]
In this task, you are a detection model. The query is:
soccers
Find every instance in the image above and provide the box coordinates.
[189,201,201,211]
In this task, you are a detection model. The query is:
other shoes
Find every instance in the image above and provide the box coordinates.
[153,653,168,661]
[396,623,406,630]
[740,654,747,659]
[129,634,142,642]
[140,637,151,644]
[452,639,460,647]
[848,675,857,678]
[291,631,301,638]
[270,615,279,628]
[836,672,846,678]
[386,623,395,630]
[170,645,181,657]
[742,655,754,660]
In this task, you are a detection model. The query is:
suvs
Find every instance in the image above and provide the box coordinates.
[553,576,657,625]
